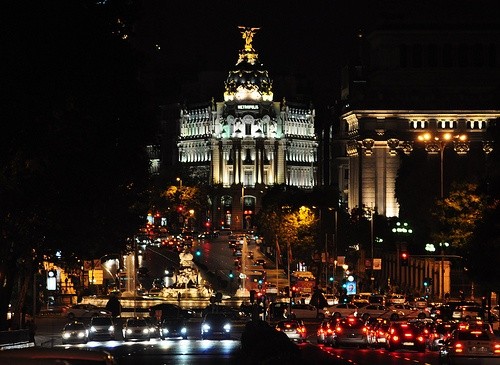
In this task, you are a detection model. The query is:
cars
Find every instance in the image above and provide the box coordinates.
[122,317,150,341]
[64,304,113,318]
[157,318,188,339]
[36,231,500,365]
[199,313,231,340]
[89,316,115,339]
[61,322,89,343]
[147,302,191,321]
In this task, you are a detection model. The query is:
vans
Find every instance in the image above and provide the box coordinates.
[0,347,116,365]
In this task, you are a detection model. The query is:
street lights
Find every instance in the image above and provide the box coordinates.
[311,206,322,234]
[329,207,338,261]
[418,132,468,258]
